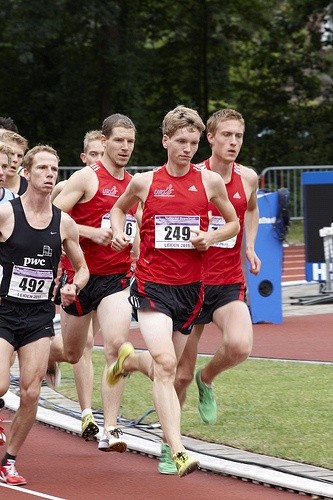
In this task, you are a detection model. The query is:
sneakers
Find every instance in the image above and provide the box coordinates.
[81,413,99,439]
[0,418,7,443]
[172,448,199,477]
[196,369,217,424]
[106,342,136,386]
[98,427,127,453]
[0,457,27,485]
[46,338,61,388]
[158,443,178,474]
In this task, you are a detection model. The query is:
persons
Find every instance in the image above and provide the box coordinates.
[0,119,21,409]
[49,130,109,440]
[40,112,146,453]
[172,108,263,426]
[0,147,91,487]
[0,116,30,196]
[106,104,241,477]
